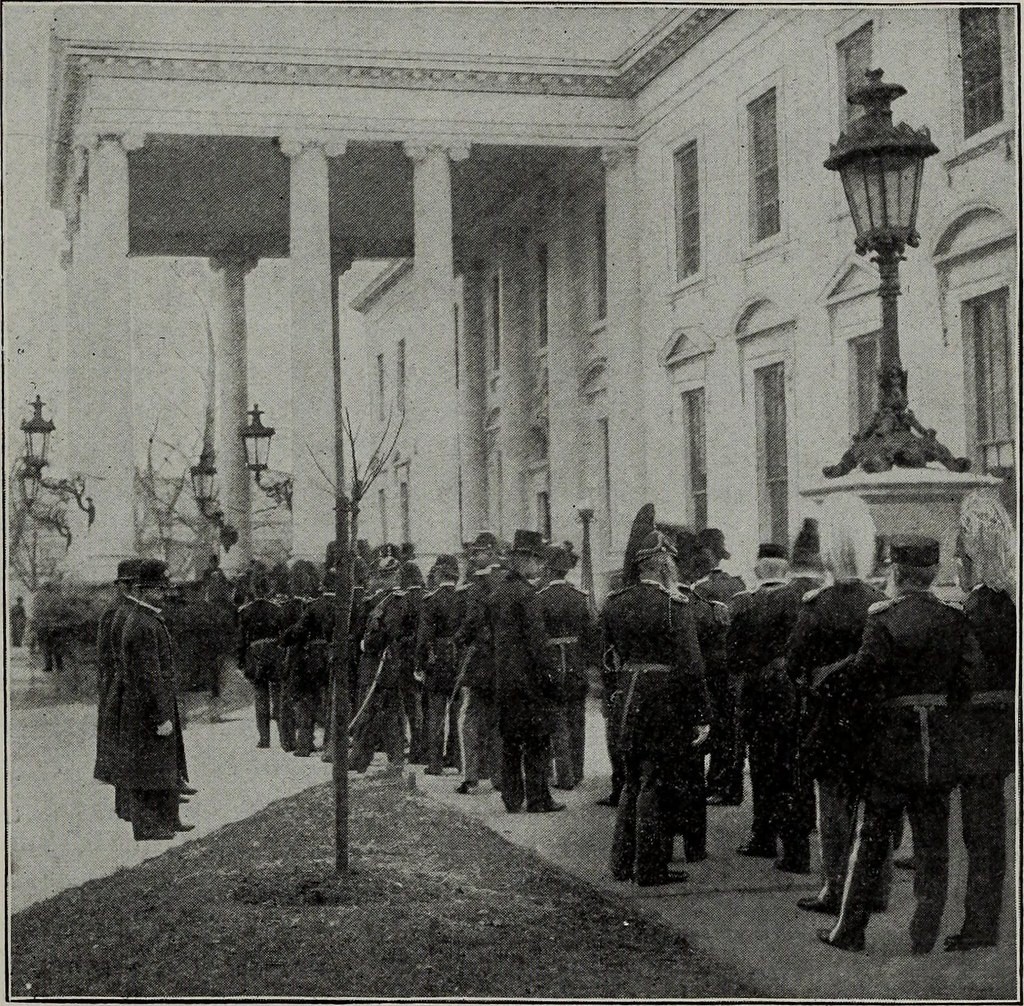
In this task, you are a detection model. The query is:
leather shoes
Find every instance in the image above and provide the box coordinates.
[943,934,997,952]
[893,855,917,871]
[637,868,692,887]
[796,895,841,917]
[736,841,778,858]
[705,791,741,806]
[687,850,709,864]
[595,796,618,808]
[815,927,865,952]
[456,780,480,795]
[527,800,567,812]
[773,858,811,874]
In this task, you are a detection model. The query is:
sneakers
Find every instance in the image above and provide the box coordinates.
[135,828,175,840]
[181,785,199,795]
[179,795,190,804]
[171,823,195,831]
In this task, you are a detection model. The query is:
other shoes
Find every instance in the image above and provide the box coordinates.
[549,774,581,790]
[385,762,405,772]
[423,766,444,776]
[442,762,463,774]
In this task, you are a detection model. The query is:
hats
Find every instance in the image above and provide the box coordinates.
[632,528,679,565]
[508,527,545,559]
[697,528,732,561]
[758,542,789,560]
[540,546,573,572]
[113,558,144,585]
[468,532,498,551]
[134,558,173,588]
[888,532,942,568]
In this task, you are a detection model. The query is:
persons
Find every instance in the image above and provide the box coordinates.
[599,502,690,888]
[537,546,589,790]
[941,489,1016,953]
[38,582,64,672]
[731,542,793,860]
[785,492,893,917]
[671,578,731,863]
[597,704,625,808]
[9,595,28,648]
[59,524,509,840]
[498,527,566,813]
[701,526,747,807]
[816,535,981,956]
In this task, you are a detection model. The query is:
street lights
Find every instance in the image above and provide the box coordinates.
[810,66,975,477]
